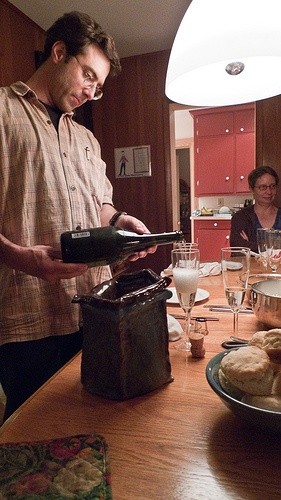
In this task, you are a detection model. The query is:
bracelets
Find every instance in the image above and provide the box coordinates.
[108,211,128,227]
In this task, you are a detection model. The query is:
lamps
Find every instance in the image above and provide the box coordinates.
[164,0,281,107]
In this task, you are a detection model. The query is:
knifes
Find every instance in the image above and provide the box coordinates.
[170,314,219,321]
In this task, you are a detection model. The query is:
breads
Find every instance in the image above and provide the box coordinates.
[219,328,281,411]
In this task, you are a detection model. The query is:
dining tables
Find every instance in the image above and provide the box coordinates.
[0,260,281,500]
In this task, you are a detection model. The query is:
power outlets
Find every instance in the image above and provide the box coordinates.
[218,198,224,207]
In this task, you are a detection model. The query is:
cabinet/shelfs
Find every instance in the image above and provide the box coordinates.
[193,220,231,261]
[189,95,281,197]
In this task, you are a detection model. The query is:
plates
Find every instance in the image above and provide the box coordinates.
[262,248,281,258]
[166,286,210,304]
[167,262,244,276]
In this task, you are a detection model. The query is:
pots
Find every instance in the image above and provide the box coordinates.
[250,280,281,328]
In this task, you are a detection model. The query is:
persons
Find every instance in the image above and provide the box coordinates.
[230,166,281,257]
[0,11,158,431]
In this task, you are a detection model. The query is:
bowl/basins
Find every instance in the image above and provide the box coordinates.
[205,347,281,422]
[219,206,230,214]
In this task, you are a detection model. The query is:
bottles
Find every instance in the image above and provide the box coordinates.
[60,225,184,268]
[244,200,249,209]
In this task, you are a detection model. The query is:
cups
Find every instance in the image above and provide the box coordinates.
[173,243,199,249]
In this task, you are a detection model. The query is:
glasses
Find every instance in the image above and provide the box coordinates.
[75,55,103,101]
[252,184,280,191]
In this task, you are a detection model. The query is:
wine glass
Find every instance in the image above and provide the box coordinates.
[171,248,200,352]
[256,227,281,278]
[220,246,250,343]
